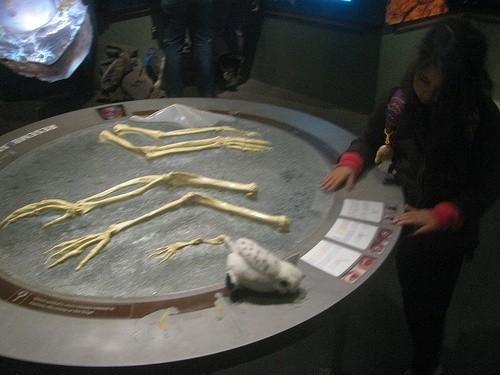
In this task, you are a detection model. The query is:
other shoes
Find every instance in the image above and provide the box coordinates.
[221,69,250,89]
[403,365,442,375]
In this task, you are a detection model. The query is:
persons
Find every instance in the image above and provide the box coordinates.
[320,17,500,375]
[154,0,267,99]
[0,0,100,110]
